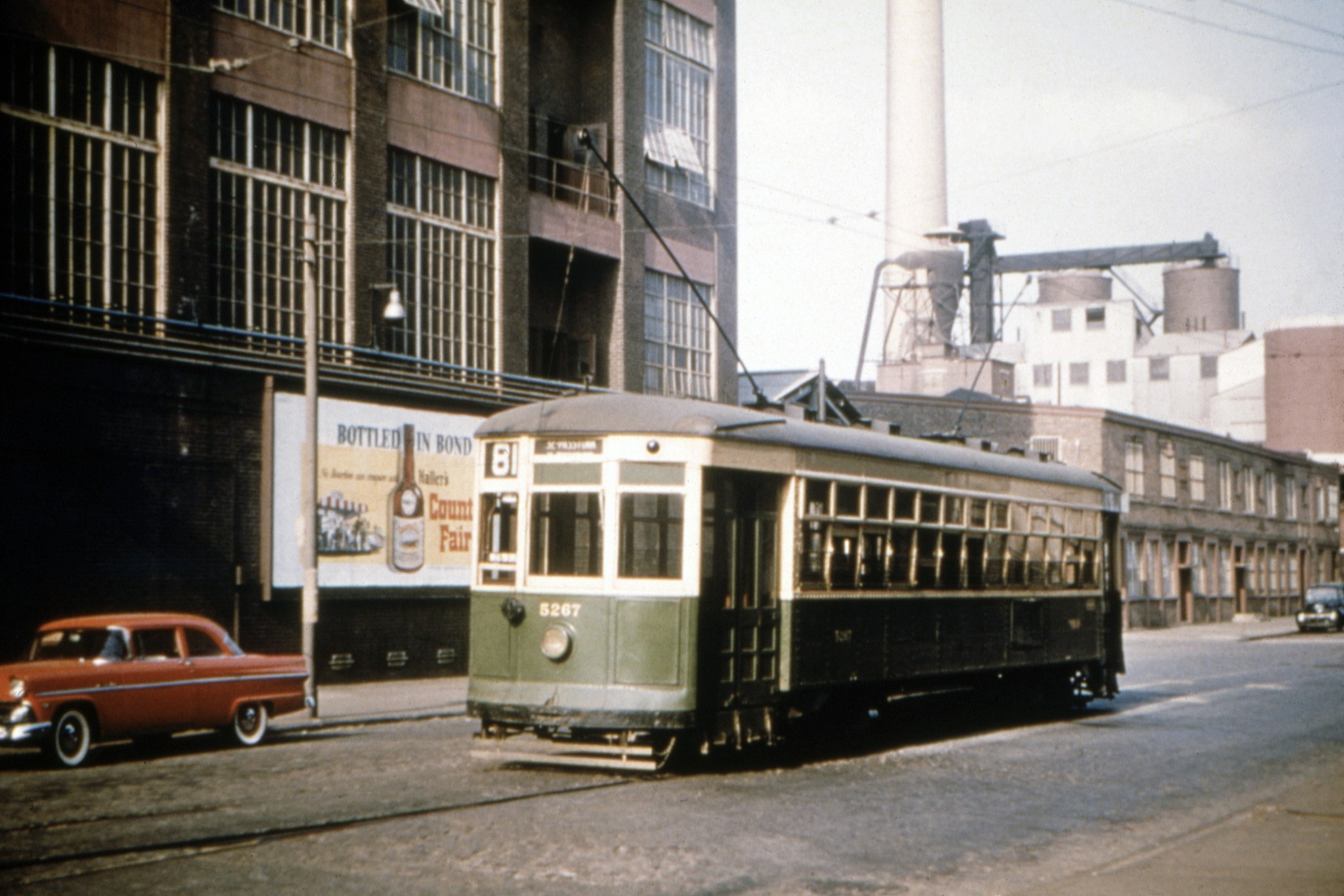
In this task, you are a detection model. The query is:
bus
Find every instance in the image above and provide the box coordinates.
[461,127,1129,775]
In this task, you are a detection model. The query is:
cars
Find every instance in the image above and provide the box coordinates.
[1,611,317,773]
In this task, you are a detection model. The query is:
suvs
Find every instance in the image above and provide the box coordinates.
[1297,581,1344,634]
